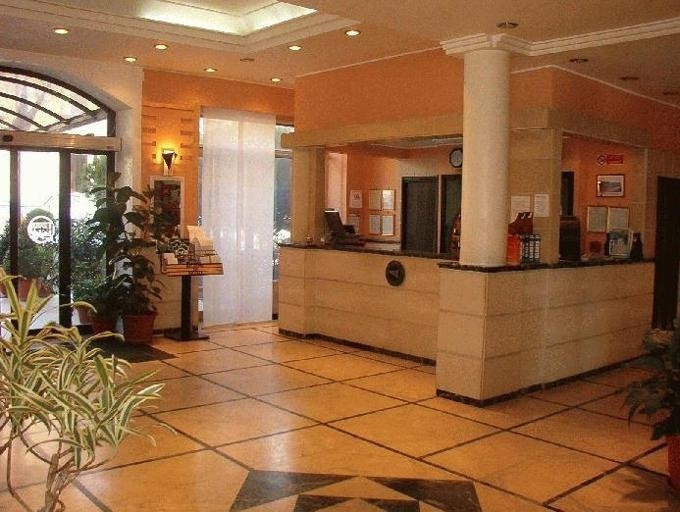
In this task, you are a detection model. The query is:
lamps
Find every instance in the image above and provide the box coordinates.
[162,148,179,175]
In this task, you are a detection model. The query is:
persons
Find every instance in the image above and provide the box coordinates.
[612,237,626,254]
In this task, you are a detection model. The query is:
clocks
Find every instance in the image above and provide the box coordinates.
[449,148,462,167]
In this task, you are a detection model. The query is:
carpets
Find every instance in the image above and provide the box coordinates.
[62,339,179,363]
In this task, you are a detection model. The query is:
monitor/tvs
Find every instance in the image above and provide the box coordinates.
[325,209,346,247]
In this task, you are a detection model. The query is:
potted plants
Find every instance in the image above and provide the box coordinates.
[615,310,680,494]
[1,207,58,302]
[70,170,176,345]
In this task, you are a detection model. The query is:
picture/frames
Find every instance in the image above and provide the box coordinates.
[367,188,395,236]
[584,205,630,233]
[595,174,625,197]
